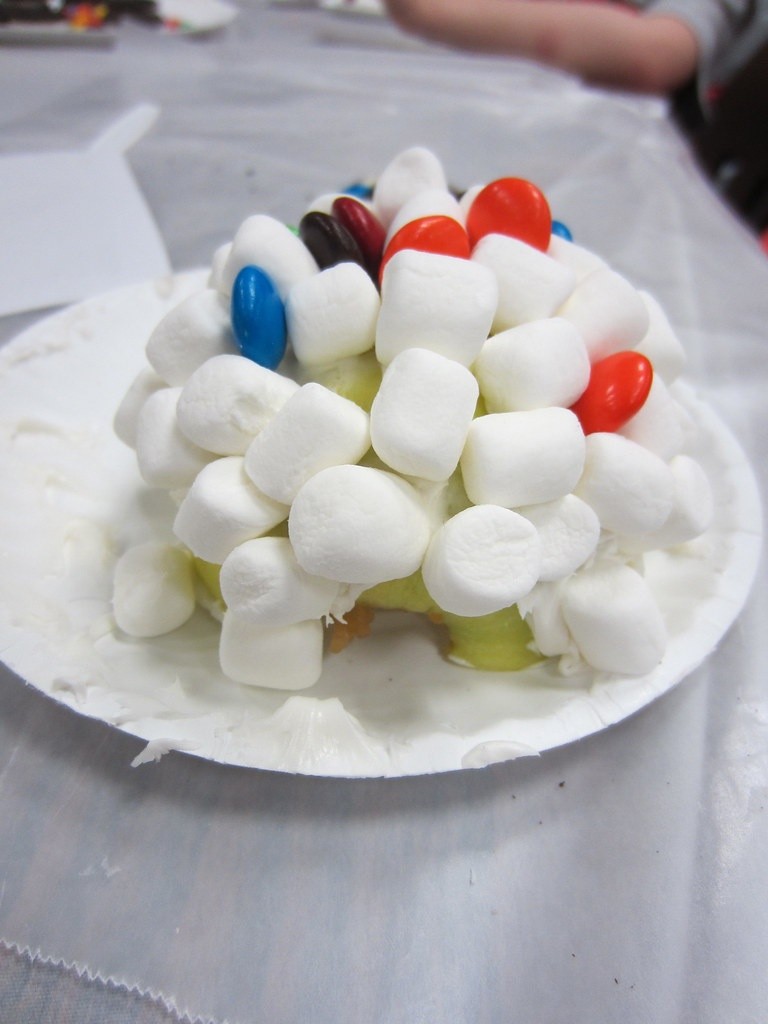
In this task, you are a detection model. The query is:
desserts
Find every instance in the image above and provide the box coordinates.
[114,148,716,688]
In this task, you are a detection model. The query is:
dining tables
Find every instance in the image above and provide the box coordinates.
[0,0,768,1023]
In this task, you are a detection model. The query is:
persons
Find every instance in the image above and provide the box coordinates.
[383,0,768,106]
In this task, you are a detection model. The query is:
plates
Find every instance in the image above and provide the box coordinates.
[1,268,762,778]
